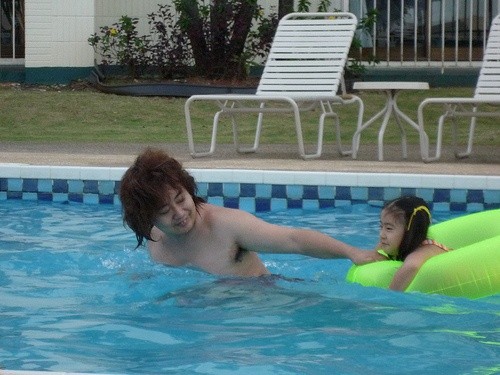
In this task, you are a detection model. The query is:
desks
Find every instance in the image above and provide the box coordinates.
[352,81,430,161]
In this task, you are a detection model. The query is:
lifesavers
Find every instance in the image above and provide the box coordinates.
[347,208,500,300]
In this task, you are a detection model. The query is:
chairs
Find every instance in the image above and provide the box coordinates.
[417,14,500,163]
[185,12,364,160]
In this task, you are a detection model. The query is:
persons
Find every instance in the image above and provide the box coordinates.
[119,149,389,278]
[374,194,456,293]
